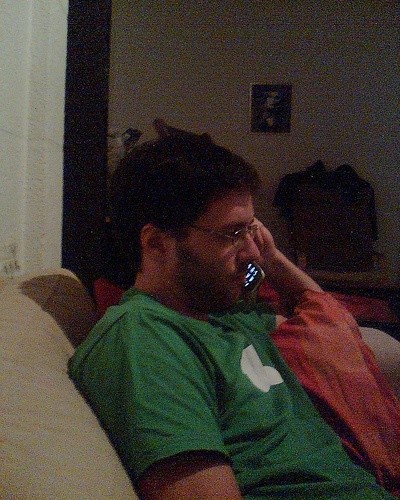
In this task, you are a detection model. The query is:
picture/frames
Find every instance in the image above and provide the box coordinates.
[248,83,292,133]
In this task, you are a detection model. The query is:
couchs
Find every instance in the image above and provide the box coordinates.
[0,268,400,499]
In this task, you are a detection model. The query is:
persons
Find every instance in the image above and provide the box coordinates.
[67,132,399,499]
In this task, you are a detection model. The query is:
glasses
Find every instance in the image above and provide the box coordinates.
[185,222,259,248]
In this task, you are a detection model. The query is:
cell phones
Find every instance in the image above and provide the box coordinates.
[242,261,266,293]
